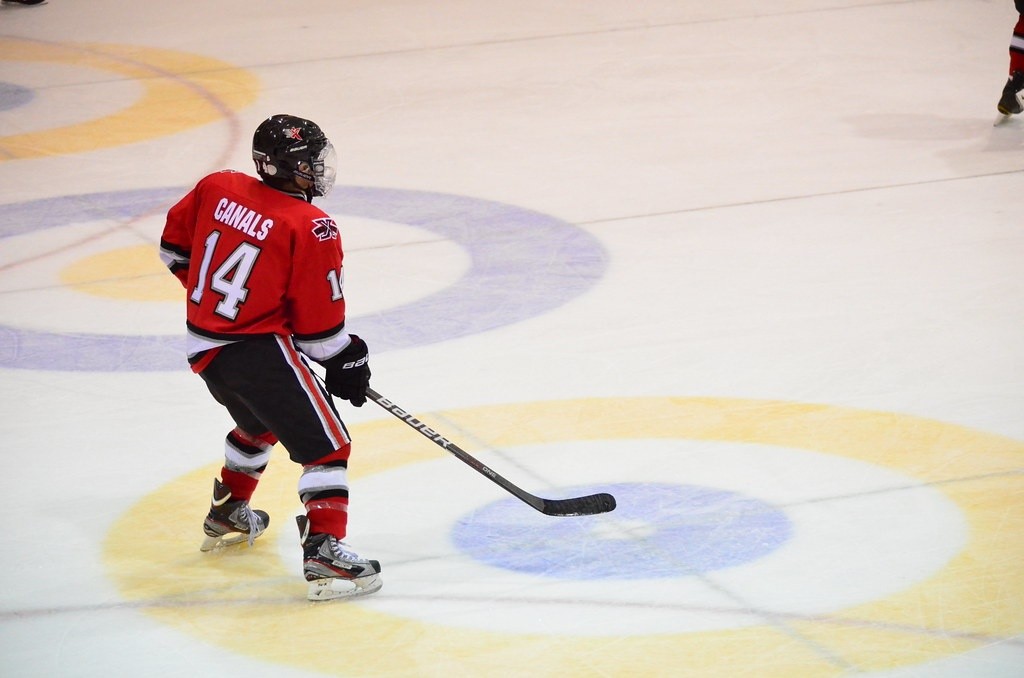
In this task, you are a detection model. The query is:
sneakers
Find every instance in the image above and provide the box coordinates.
[296,516,382,601]
[200,477,269,552]
[993,71,1024,126]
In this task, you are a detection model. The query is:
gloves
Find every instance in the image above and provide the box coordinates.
[321,334,370,407]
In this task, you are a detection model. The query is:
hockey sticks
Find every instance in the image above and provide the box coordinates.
[365,388,617,516]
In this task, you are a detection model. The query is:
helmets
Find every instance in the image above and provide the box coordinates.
[252,114,328,201]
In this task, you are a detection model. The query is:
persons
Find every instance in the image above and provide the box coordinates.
[157,113,383,602]
[992,0,1024,127]
